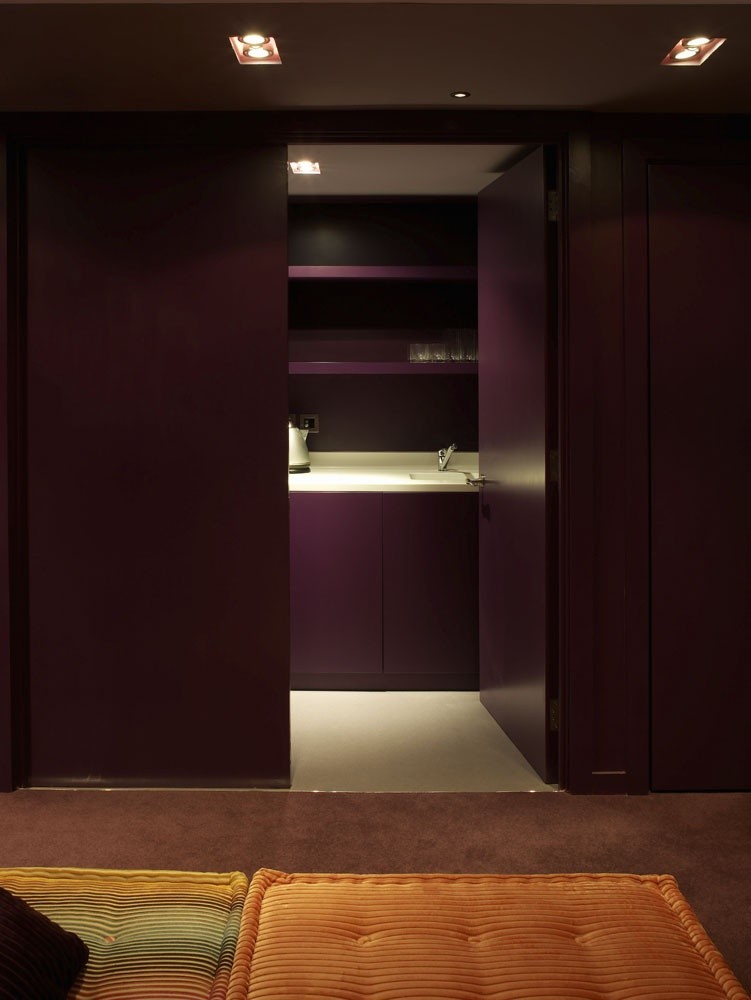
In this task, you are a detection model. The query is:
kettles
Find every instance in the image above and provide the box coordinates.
[288,420,311,472]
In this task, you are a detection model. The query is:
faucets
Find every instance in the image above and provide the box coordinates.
[437,443,457,471]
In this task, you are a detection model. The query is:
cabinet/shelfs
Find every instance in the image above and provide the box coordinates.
[285,265,480,378]
[288,489,482,694]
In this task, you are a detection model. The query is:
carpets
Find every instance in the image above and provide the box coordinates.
[1,786,751,1000]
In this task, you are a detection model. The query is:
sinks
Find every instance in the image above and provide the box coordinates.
[408,472,467,483]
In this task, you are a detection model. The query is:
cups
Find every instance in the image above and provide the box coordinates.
[460,343,476,361]
[444,343,461,361]
[430,343,444,362]
[410,344,429,363]
